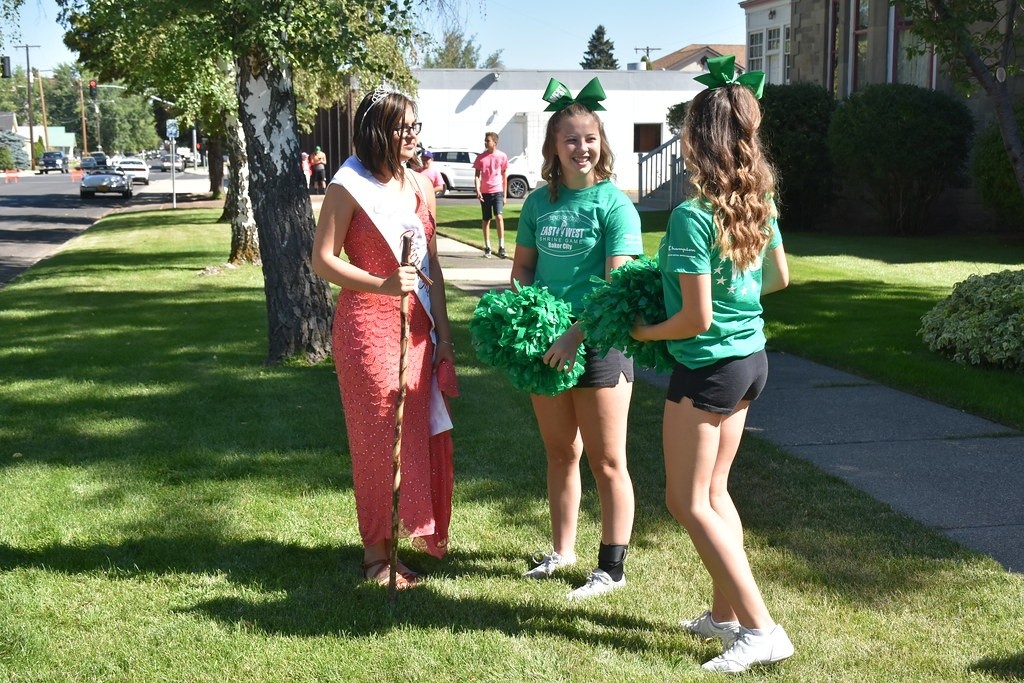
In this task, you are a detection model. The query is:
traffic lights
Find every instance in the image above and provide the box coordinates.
[90,80,97,99]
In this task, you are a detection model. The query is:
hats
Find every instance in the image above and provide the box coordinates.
[301,152,310,157]
[420,151,433,158]
[315,146,321,154]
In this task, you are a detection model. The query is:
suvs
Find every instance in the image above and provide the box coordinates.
[421,146,537,199]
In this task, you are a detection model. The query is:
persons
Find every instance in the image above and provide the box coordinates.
[472,132,509,259]
[311,87,459,589]
[412,149,446,193]
[509,103,645,602]
[310,145,327,195]
[301,152,312,194]
[626,82,795,671]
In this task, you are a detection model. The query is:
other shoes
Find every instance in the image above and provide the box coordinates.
[498,247,508,258]
[523,544,577,580]
[484,247,492,258]
[567,568,627,597]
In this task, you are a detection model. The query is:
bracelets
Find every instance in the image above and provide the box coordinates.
[439,339,454,346]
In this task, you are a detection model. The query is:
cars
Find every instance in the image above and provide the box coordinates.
[81,156,98,170]
[89,149,109,169]
[79,164,133,199]
[38,150,69,174]
[114,156,153,185]
[110,148,197,172]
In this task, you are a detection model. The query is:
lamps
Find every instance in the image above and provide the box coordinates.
[492,72,500,81]
[768,9,776,20]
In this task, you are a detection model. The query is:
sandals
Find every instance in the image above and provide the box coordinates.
[363,559,410,590]
[388,557,426,582]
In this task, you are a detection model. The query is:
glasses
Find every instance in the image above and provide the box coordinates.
[394,123,422,138]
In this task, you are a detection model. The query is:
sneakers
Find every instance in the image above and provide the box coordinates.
[702,625,794,674]
[682,611,741,646]
